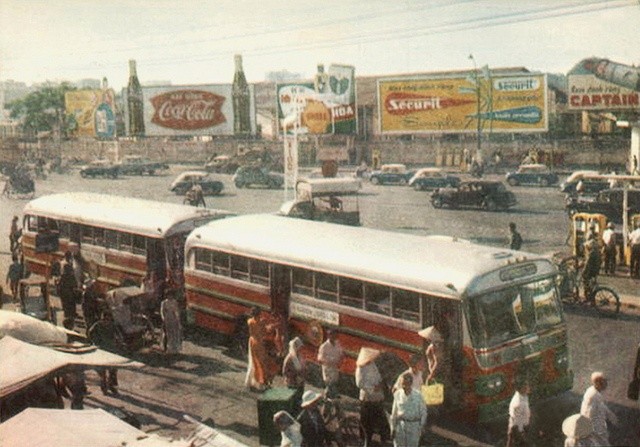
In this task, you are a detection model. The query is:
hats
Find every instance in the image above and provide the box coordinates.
[583,239,601,248]
[417,325,445,342]
[562,412,594,439]
[356,347,379,366]
[301,388,321,407]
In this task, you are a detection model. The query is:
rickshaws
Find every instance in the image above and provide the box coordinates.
[87,285,154,350]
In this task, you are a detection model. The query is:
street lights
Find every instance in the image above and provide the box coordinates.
[468,53,480,159]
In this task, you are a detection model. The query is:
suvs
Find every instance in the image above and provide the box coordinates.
[567,188,640,219]
[120,156,159,174]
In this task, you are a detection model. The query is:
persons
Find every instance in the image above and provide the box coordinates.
[6,252,21,302]
[320,195,342,211]
[504,379,534,445]
[589,226,598,242]
[79,279,99,340]
[389,374,428,447]
[598,151,608,174]
[562,412,596,445]
[1,143,77,197]
[392,353,426,400]
[120,273,139,286]
[492,148,502,173]
[250,157,264,178]
[577,191,594,210]
[260,305,283,360]
[317,329,344,418]
[9,214,21,252]
[608,167,617,184]
[59,251,81,316]
[602,221,617,274]
[356,159,367,176]
[244,305,280,392]
[59,252,79,287]
[580,370,620,446]
[583,239,603,305]
[281,331,307,389]
[88,279,104,320]
[629,222,640,280]
[183,184,205,209]
[274,411,303,447]
[354,345,395,447]
[88,307,123,397]
[610,172,620,189]
[144,271,163,313]
[508,222,522,251]
[159,288,184,356]
[564,210,577,245]
[521,144,542,166]
[419,324,448,427]
[297,389,328,445]
[461,144,470,173]
[575,178,585,194]
[259,145,270,167]
[632,154,639,176]
[471,157,482,179]
[170,268,185,312]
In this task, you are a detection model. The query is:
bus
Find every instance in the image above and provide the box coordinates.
[12,191,236,313]
[184,214,573,423]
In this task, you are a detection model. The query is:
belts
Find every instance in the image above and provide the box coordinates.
[402,418,418,423]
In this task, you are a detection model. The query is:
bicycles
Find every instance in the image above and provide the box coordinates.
[324,397,367,446]
[563,265,619,315]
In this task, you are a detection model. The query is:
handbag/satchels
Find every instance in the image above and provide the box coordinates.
[421,377,444,406]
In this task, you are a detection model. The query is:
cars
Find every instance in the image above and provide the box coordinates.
[170,170,222,194]
[80,161,118,177]
[506,164,557,185]
[370,163,412,185]
[429,180,516,211]
[234,165,284,188]
[408,169,460,190]
[207,156,235,173]
[561,171,609,191]
